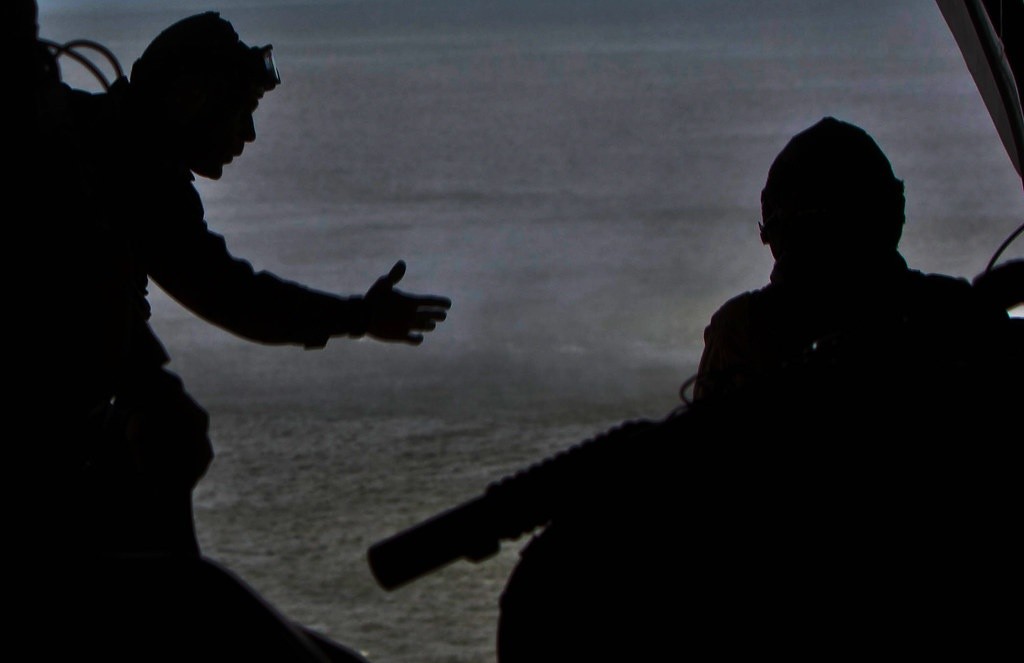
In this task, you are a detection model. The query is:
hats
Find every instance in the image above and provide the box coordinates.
[130,9,273,147]
[757,116,906,260]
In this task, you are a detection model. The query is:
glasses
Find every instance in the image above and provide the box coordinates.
[248,42,281,87]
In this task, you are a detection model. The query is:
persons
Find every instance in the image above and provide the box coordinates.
[2,7,453,663]
[681,114,1024,663]
